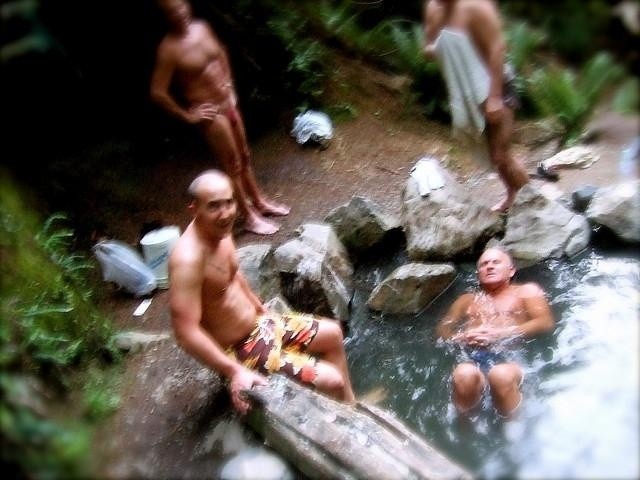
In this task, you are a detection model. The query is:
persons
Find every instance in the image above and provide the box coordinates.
[434,248,554,421]
[422,0,531,212]
[149,0,291,235]
[168,169,354,415]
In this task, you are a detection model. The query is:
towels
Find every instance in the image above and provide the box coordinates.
[435,29,490,138]
